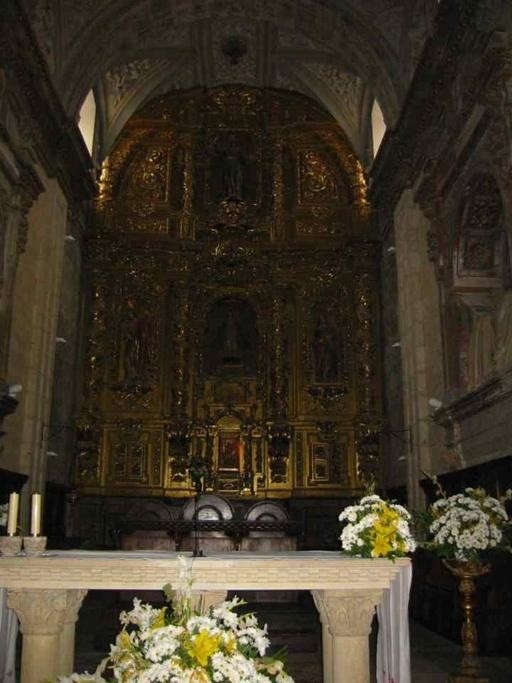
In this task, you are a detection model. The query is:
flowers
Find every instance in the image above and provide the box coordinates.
[55,554,296,682]
[334,493,419,565]
[421,467,512,572]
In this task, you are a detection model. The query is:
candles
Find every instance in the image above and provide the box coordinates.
[6,492,19,534]
[31,492,42,534]
